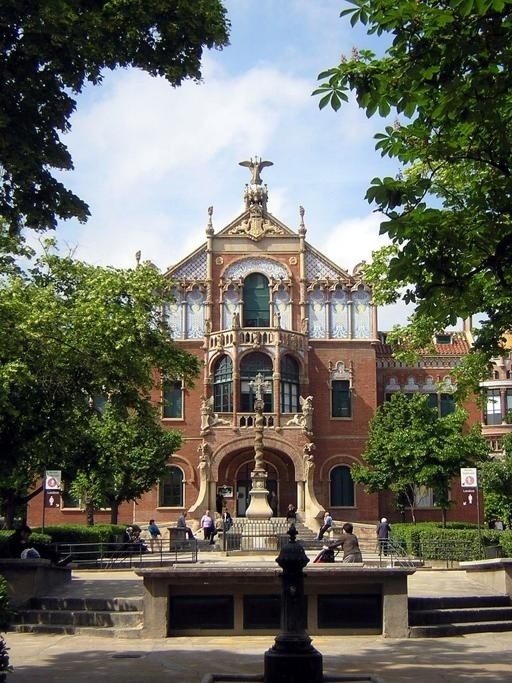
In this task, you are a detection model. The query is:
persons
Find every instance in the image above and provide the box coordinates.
[216,489,224,513]
[375,517,392,556]
[124,526,150,553]
[200,509,213,540]
[148,519,163,553]
[314,511,333,540]
[1,524,74,567]
[208,512,223,545]
[131,530,148,546]
[322,522,363,563]
[222,507,232,531]
[287,503,297,523]
[176,509,195,540]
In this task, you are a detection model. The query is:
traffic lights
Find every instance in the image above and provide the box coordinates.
[461,489,475,508]
[46,490,61,509]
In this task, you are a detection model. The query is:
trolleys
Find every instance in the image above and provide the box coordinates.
[312,545,340,563]
